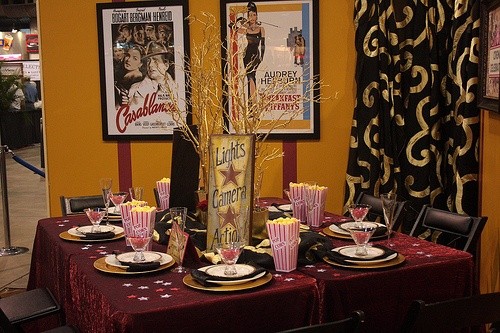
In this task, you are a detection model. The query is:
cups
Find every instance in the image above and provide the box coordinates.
[128,187,143,201]
[284,189,292,200]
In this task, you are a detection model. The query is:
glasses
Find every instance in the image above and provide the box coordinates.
[146,29,152,31]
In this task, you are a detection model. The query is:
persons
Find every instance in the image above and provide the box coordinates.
[113,23,175,108]
[22,77,38,111]
[5,83,26,110]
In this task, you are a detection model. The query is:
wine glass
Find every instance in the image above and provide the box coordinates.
[83,208,105,232]
[380,193,397,248]
[109,192,129,214]
[348,203,370,228]
[170,207,190,272]
[347,222,379,257]
[101,179,114,226]
[127,232,153,260]
[216,242,244,275]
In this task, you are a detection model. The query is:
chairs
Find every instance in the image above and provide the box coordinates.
[0,188,500,333]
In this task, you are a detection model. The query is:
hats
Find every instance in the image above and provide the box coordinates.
[119,24,130,32]
[143,41,169,59]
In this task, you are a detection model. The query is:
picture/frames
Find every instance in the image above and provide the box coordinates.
[220,0,320,140]
[475,0,500,113]
[97,0,190,141]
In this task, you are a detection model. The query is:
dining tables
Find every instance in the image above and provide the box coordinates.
[27,197,473,333]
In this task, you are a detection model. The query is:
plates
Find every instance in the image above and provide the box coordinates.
[328,220,386,236]
[197,263,266,285]
[268,205,292,211]
[102,207,127,221]
[67,226,123,238]
[93,257,176,274]
[323,227,387,240]
[105,252,172,269]
[332,245,397,263]
[324,254,405,268]
[58,230,125,242]
[182,273,273,291]
